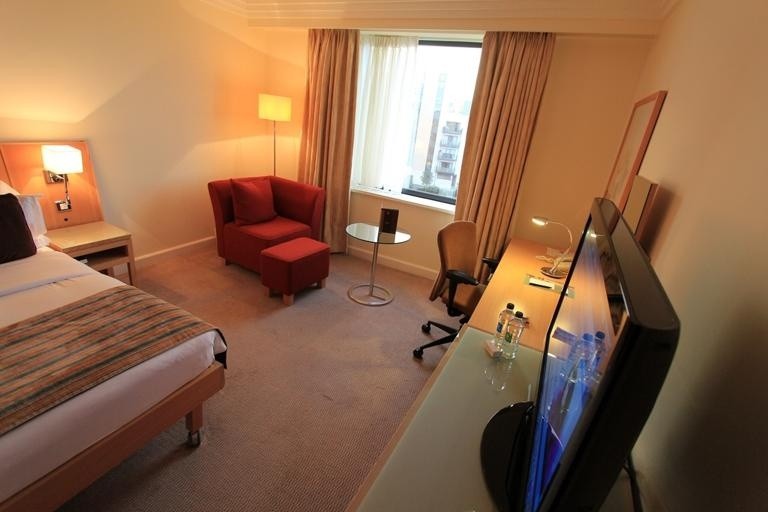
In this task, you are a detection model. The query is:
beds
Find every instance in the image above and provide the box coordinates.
[0,179,228,512]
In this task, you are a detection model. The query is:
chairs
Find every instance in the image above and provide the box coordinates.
[208,175,327,277]
[412,220,500,360]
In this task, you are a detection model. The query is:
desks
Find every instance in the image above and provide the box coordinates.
[344,237,641,511]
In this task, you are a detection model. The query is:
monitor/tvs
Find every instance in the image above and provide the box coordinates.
[479,195,682,512]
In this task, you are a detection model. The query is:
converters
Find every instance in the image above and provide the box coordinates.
[484,339,504,358]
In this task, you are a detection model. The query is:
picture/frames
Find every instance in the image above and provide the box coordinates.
[603,89,668,215]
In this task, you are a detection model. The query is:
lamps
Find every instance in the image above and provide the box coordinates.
[531,213,574,279]
[41,144,84,207]
[258,93,291,177]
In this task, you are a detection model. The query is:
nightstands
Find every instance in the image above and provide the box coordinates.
[45,220,137,289]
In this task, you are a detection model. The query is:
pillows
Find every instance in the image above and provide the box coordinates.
[228,175,278,226]
[0,193,48,264]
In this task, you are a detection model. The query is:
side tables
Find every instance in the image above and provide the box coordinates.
[345,221,412,306]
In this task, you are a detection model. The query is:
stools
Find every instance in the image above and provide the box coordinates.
[260,237,330,306]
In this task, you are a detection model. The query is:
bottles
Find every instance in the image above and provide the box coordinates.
[559,328,606,385]
[483,359,513,393]
[494,303,515,350]
[500,311,525,360]
